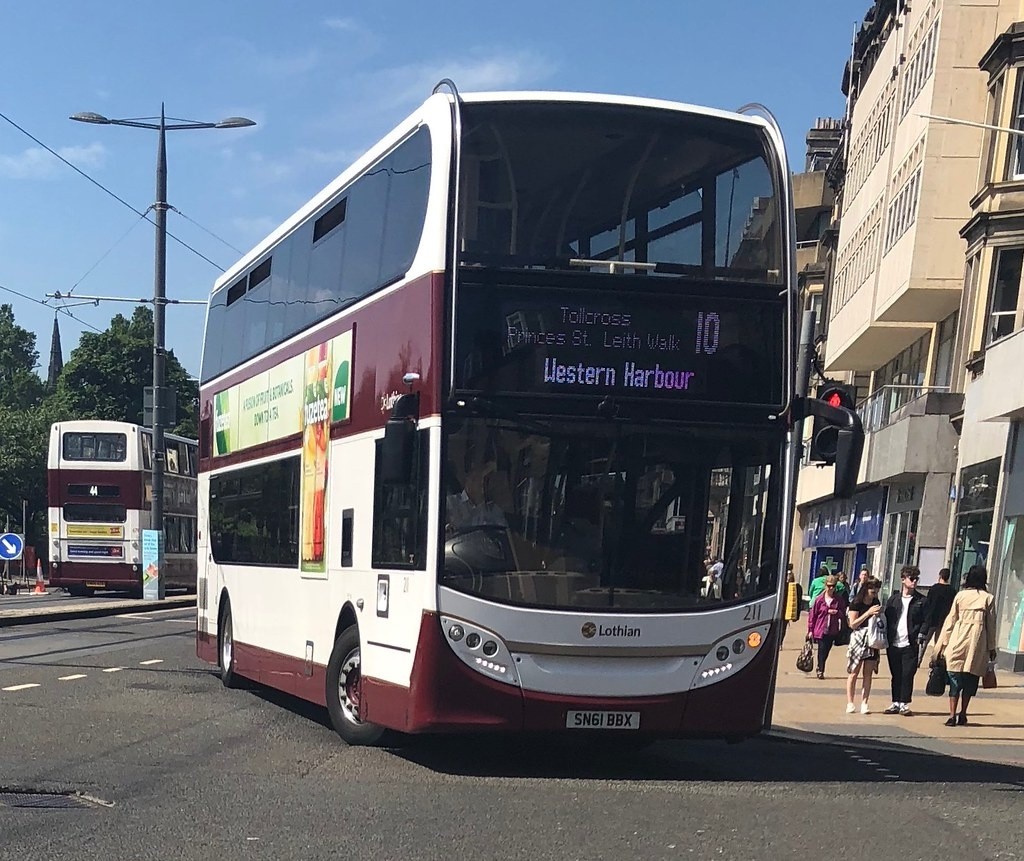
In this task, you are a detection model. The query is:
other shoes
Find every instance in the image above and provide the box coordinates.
[816,668,824,679]
[899,704,912,715]
[945,717,956,726]
[884,703,900,714]
[861,704,871,714]
[958,714,967,723]
[846,703,855,713]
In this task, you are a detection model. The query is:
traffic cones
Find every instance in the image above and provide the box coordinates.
[29,558,50,596]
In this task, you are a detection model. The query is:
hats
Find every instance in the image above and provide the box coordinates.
[826,575,837,586]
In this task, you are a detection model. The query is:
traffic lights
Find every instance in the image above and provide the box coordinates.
[810,384,858,463]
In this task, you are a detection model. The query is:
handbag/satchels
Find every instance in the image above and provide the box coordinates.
[834,616,853,646]
[868,613,890,649]
[796,637,813,672]
[983,660,998,688]
[926,654,948,696]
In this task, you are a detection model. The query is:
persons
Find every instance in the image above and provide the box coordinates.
[846,576,884,714]
[883,565,931,716]
[935,565,997,728]
[917,568,956,668]
[848,569,869,603]
[445,467,505,534]
[808,575,848,680]
[809,567,849,609]
[737,558,758,583]
[700,555,724,598]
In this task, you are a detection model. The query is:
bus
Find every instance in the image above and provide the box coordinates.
[198,77,867,762]
[47,421,199,597]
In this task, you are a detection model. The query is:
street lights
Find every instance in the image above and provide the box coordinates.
[66,101,256,531]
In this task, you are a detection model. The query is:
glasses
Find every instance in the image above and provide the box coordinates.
[906,575,919,582]
[865,584,880,590]
